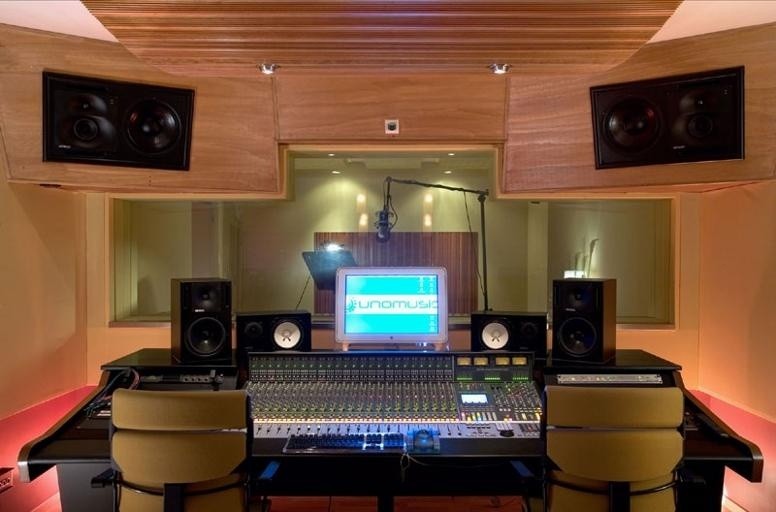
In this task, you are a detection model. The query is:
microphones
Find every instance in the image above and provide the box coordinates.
[376,210,391,243]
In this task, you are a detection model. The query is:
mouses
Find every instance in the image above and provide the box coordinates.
[414,429,433,450]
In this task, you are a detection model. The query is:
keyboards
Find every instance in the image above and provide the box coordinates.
[282,432,404,454]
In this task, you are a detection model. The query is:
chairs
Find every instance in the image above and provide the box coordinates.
[520,382,683,512]
[90,388,270,511]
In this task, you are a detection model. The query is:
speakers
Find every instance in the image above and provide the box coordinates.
[552,278,617,364]
[235,310,312,362]
[170,277,232,363]
[590,67,746,170]
[41,70,196,171]
[471,312,548,359]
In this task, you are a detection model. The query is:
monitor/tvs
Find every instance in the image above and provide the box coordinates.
[338,265,447,344]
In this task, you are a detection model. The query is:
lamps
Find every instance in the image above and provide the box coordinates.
[256,63,280,75]
[487,64,513,75]
